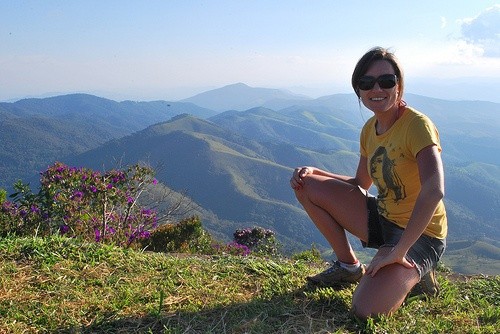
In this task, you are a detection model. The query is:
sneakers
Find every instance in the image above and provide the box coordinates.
[410,269,439,296]
[308,260,368,283]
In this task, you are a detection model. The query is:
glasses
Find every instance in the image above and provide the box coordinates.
[356,74,398,90]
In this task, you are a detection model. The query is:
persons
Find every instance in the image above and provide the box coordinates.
[288,47,448,325]
